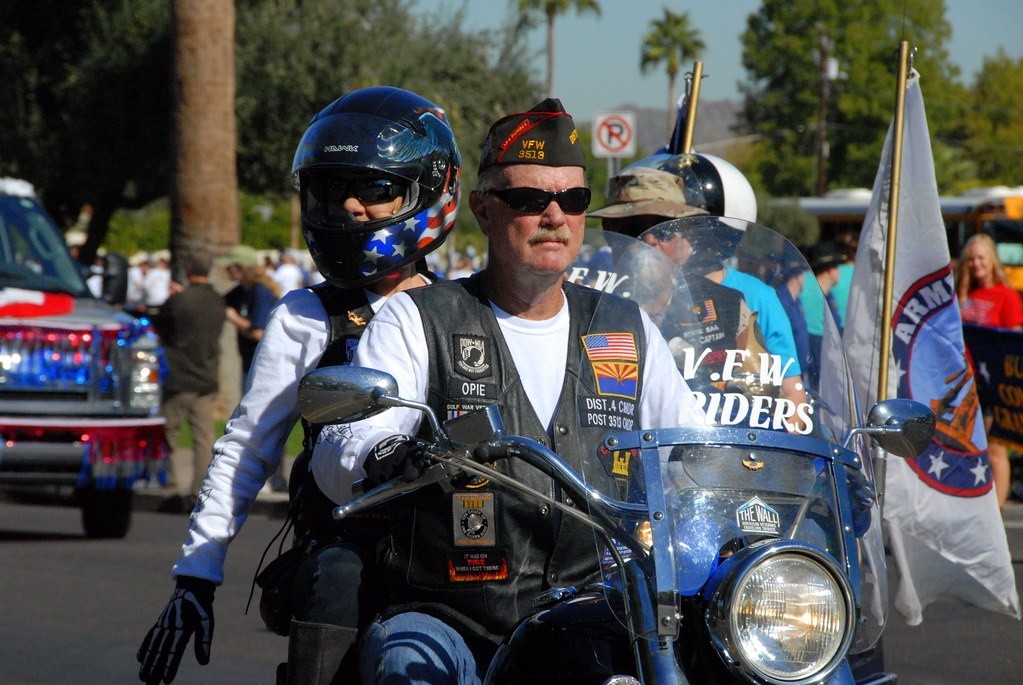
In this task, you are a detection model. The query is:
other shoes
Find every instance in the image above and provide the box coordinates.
[151,494,199,515]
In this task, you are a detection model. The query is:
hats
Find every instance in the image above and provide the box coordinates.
[584,166,710,218]
[128,249,150,267]
[212,244,261,269]
[477,97,588,167]
[150,250,175,262]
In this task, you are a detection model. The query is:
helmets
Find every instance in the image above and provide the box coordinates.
[659,150,760,256]
[289,86,460,287]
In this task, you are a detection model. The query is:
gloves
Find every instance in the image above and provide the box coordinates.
[136,575,214,685]
[364,433,439,484]
[841,466,871,539]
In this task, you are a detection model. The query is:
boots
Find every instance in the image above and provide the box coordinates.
[285,617,359,685]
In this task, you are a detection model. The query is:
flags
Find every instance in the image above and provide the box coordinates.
[839,61,1023,625]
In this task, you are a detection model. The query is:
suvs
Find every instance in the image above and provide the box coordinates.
[0,176,172,542]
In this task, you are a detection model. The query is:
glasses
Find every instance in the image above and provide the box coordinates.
[607,218,686,243]
[307,170,411,205]
[485,186,592,214]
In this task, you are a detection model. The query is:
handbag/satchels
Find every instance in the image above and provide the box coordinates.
[255,545,314,636]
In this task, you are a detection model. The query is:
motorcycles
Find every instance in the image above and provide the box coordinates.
[298,216,937,685]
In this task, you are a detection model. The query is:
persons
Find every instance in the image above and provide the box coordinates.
[423,243,488,281]
[590,143,816,437]
[311,96,718,685]
[135,83,461,685]
[951,232,1023,514]
[90,242,331,515]
[564,236,616,292]
[740,222,859,390]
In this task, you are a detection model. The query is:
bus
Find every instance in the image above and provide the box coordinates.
[756,184,1023,298]
